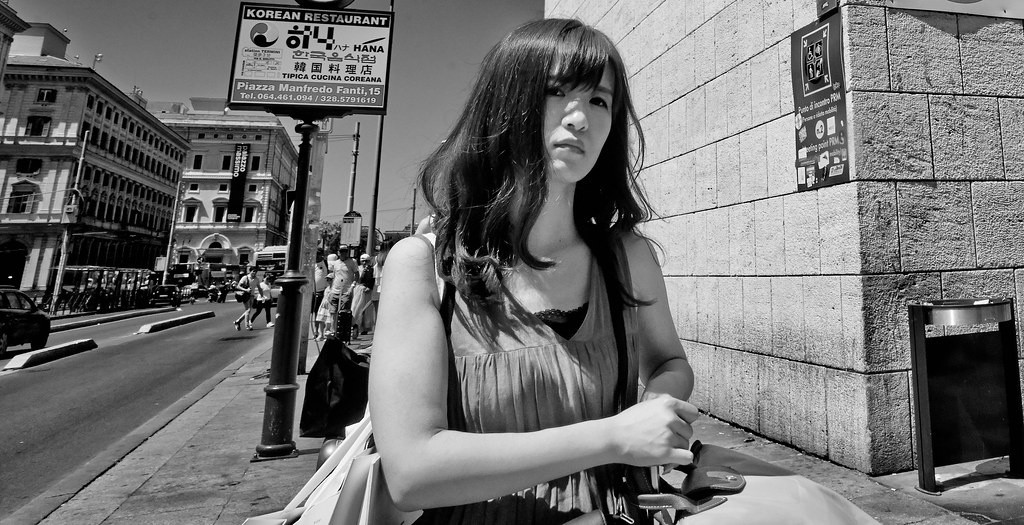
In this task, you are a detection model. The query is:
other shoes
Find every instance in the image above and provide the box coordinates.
[314,334,324,342]
[361,330,368,335]
[352,328,360,340]
[266,322,274,328]
[246,325,250,331]
[249,320,253,330]
[232,321,241,331]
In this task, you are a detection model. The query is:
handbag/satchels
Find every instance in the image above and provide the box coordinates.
[560,509,607,525]
[241,417,424,525]
[235,291,252,303]
[635,440,883,525]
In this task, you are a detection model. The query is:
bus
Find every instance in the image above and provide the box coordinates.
[171,263,247,286]
[252,246,287,302]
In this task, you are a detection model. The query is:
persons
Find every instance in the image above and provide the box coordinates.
[291,17,700,525]
[208,243,395,342]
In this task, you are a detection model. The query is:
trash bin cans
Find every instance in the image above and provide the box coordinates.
[899,296,1024,495]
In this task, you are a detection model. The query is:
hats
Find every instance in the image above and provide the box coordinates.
[326,273,334,279]
[360,254,370,261]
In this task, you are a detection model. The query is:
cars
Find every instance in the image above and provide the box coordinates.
[150,284,183,307]
[0,285,50,356]
